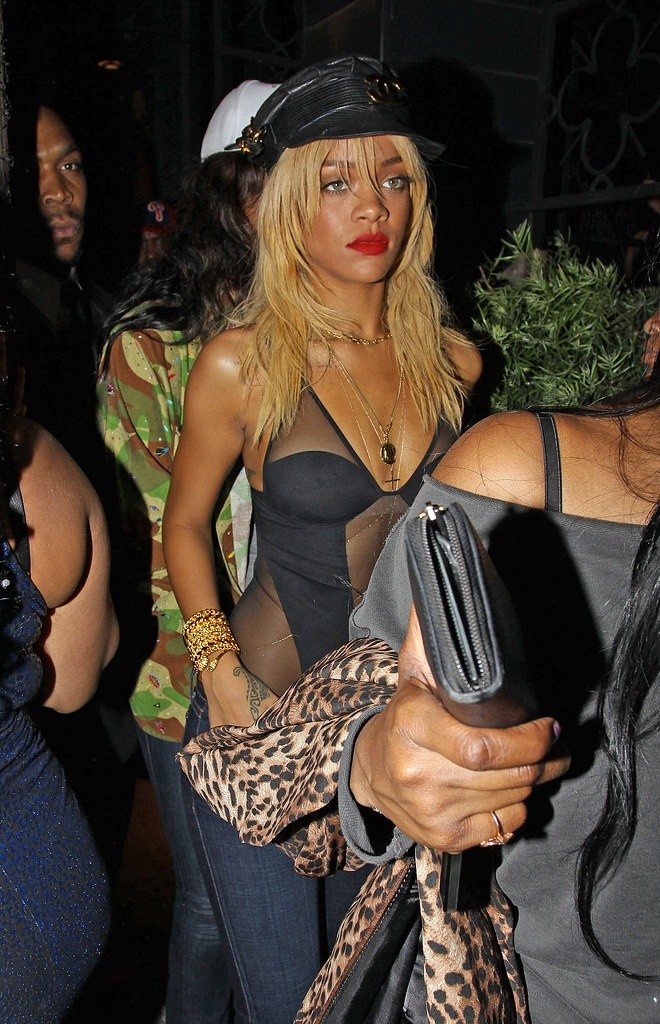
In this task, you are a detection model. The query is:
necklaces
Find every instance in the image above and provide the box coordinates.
[319,337,405,489]
[325,328,403,346]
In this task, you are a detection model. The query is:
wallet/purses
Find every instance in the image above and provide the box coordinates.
[402,502,541,730]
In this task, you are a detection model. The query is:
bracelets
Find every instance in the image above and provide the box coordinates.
[181,610,238,672]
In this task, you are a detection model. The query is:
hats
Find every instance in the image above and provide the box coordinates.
[201,79,281,164]
[235,56,445,161]
[142,201,178,236]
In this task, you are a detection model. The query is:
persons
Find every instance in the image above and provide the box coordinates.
[163,55,484,1024]
[136,198,175,264]
[337,304,660,1024]
[95,79,278,1024]
[129,90,152,259]
[0,98,122,1024]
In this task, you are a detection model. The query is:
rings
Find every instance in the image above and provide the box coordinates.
[480,811,513,847]
